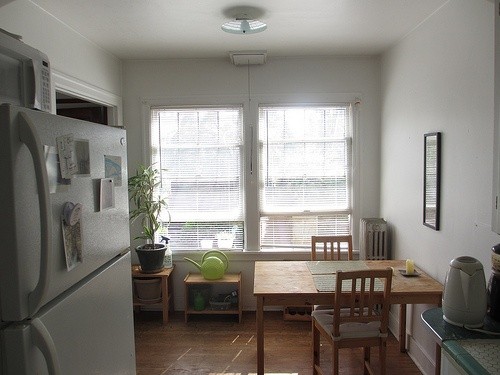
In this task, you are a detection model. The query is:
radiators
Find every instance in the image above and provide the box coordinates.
[359,217,388,261]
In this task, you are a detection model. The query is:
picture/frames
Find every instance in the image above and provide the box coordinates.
[423,132,441,231]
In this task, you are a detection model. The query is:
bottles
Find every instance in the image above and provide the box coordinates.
[487,245,500,327]
[195,292,205,311]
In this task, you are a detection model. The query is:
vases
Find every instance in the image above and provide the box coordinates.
[135,277,161,304]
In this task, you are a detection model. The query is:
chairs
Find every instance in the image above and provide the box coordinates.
[310,234,354,311]
[311,266,393,375]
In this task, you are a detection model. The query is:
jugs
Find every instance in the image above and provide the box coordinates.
[183,250,229,280]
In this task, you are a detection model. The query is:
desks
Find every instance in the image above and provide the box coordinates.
[420,304,500,375]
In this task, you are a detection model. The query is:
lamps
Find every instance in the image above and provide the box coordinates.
[221,18,267,35]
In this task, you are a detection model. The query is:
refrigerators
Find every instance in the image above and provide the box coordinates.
[0,103,135,375]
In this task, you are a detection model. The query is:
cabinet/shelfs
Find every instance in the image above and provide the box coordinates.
[131,264,174,324]
[183,271,242,328]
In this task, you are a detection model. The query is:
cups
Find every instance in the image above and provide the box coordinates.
[406,258,414,274]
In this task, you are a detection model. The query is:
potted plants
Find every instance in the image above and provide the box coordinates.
[130,161,167,273]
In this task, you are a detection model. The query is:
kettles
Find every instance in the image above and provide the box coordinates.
[441,256,488,328]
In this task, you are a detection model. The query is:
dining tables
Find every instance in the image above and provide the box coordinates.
[252,259,449,374]
